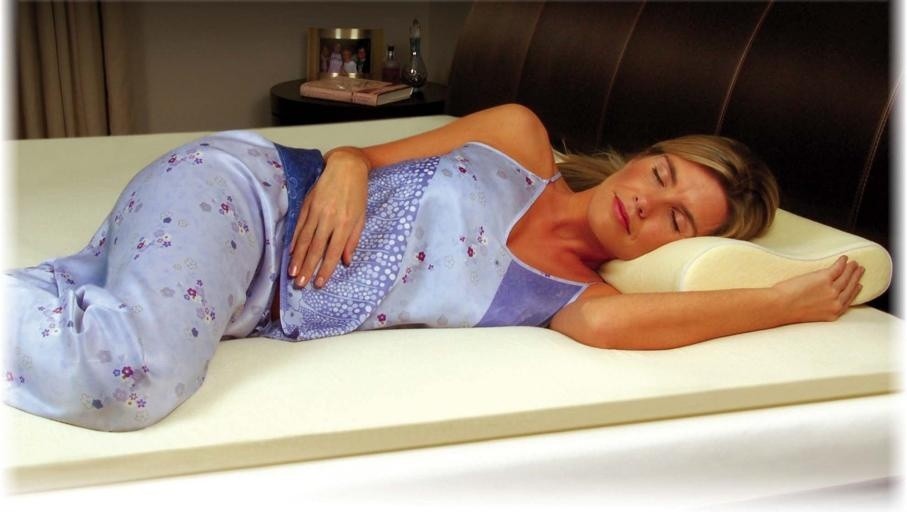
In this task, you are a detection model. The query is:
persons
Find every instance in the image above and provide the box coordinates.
[0,100,864,434]
[320,42,368,79]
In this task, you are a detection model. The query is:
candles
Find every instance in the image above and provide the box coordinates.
[409,18,421,39]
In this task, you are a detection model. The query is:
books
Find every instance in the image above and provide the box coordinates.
[299,75,414,106]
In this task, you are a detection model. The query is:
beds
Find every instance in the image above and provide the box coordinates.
[1,0,905,506]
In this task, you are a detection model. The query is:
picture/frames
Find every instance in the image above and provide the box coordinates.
[305,28,384,82]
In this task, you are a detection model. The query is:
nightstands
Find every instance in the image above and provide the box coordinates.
[269,78,449,127]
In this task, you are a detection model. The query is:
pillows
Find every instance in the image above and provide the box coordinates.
[595,205,894,307]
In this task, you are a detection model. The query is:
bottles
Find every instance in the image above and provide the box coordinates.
[401,19,428,88]
[381,44,401,84]
